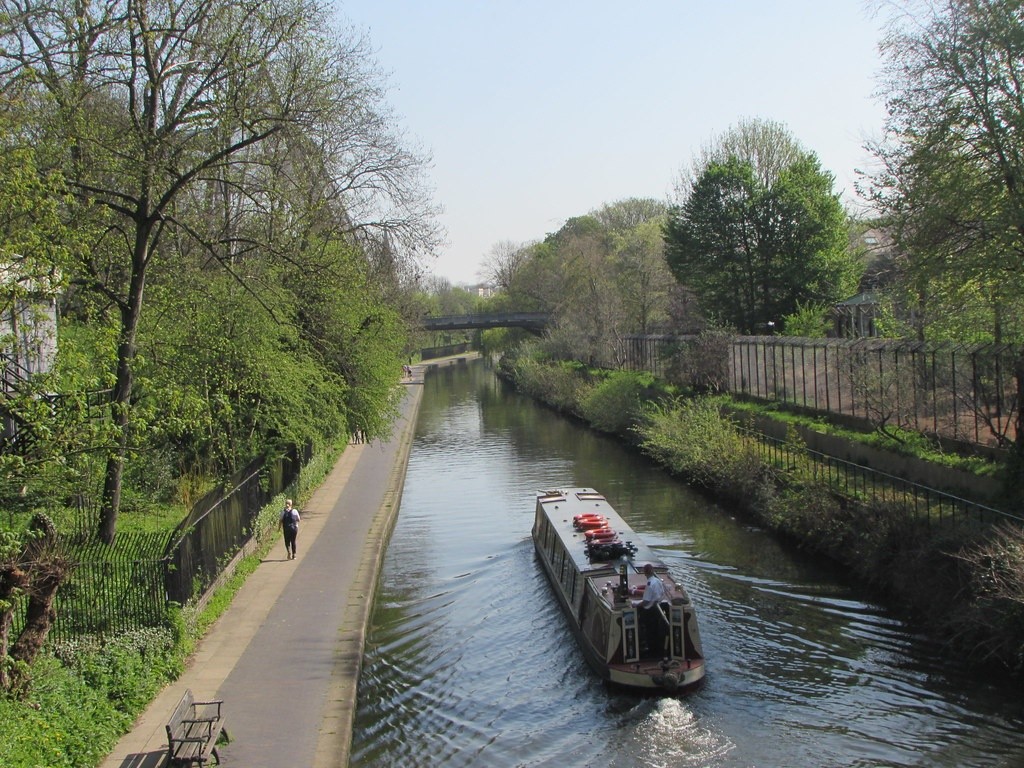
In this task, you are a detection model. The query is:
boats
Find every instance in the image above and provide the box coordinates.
[529,483,706,693]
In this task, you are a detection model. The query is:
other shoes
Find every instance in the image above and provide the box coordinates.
[287,556,292,560]
[292,555,296,560]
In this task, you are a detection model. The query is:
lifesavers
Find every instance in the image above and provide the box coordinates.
[630,584,645,596]
[574,513,621,545]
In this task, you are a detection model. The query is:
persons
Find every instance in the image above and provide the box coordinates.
[355,428,365,444]
[407,366,412,378]
[277,499,300,560]
[402,365,407,371]
[632,564,664,641]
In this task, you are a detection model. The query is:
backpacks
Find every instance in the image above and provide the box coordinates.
[282,508,296,531]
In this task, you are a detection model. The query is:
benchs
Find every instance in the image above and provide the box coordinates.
[166,688,228,768]
